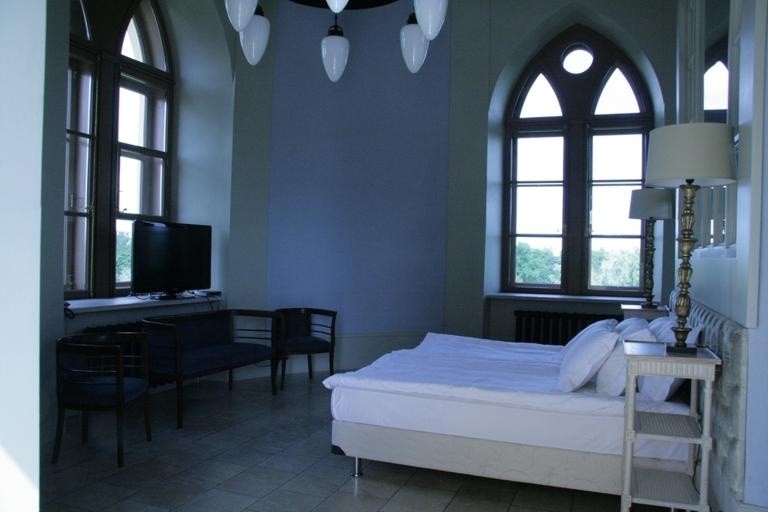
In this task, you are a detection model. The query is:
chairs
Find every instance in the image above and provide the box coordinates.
[50,322,153,469]
[275,307,337,391]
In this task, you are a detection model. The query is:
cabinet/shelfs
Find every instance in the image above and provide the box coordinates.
[617,338,722,512]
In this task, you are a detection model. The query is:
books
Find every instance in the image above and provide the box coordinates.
[193,289,222,297]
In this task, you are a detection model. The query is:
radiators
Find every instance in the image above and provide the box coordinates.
[513,310,622,350]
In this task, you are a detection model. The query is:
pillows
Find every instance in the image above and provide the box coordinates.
[554,316,702,404]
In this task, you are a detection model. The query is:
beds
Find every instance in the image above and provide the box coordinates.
[325,329,719,512]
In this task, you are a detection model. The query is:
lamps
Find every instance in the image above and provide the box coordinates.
[222,0,449,85]
[644,123,739,358]
[629,189,673,308]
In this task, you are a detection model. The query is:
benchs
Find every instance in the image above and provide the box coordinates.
[137,309,278,429]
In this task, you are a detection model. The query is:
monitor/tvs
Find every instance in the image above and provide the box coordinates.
[131,220,211,300]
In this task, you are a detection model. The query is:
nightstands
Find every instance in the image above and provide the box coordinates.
[620,303,669,322]
